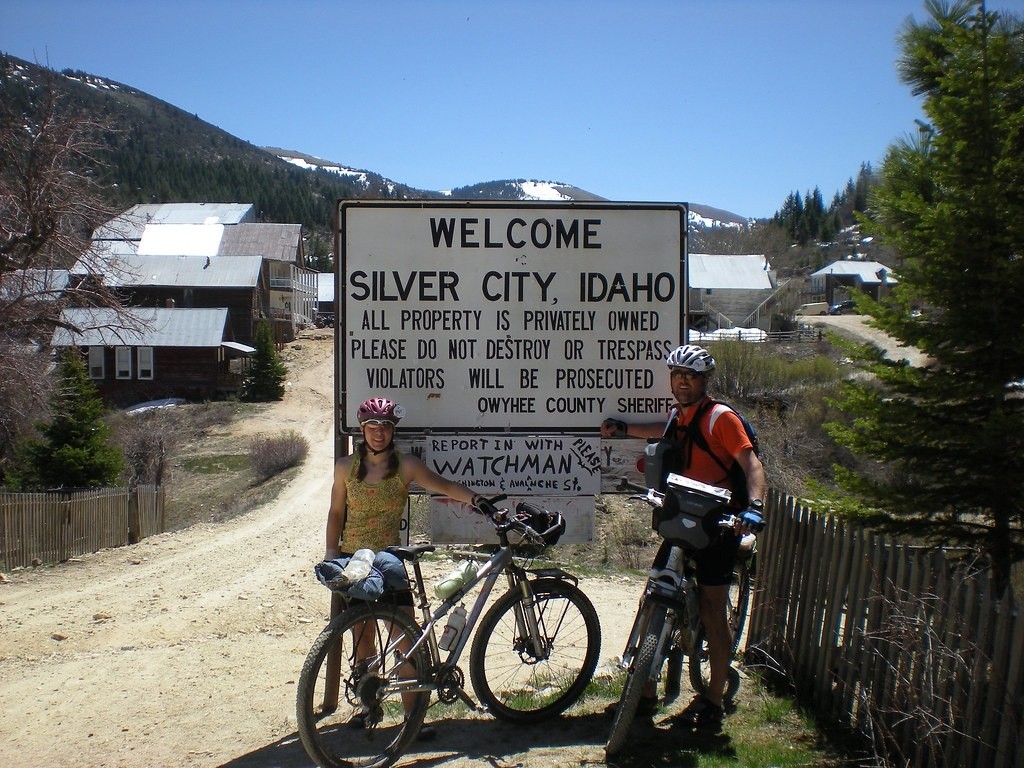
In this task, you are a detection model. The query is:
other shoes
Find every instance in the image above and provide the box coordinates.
[605,696,659,717]
[676,695,724,727]
[417,724,435,740]
[349,705,384,728]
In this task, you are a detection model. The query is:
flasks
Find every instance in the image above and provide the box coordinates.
[438,601,468,652]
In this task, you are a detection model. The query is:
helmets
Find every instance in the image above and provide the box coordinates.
[667,344,716,378]
[357,398,405,426]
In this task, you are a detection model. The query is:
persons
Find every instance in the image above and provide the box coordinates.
[601,344,765,729]
[324,397,498,740]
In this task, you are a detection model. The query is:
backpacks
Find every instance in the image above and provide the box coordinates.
[693,400,760,509]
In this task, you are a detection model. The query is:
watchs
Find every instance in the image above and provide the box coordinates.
[748,499,763,510]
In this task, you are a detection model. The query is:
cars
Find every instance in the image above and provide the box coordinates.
[828,299,861,315]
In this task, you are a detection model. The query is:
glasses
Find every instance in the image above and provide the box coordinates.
[366,423,392,431]
[671,370,700,380]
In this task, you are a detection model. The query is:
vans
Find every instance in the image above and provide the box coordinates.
[795,302,829,316]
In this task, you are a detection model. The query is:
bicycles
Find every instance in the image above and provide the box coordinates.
[602,470,766,756]
[296,493,602,768]
